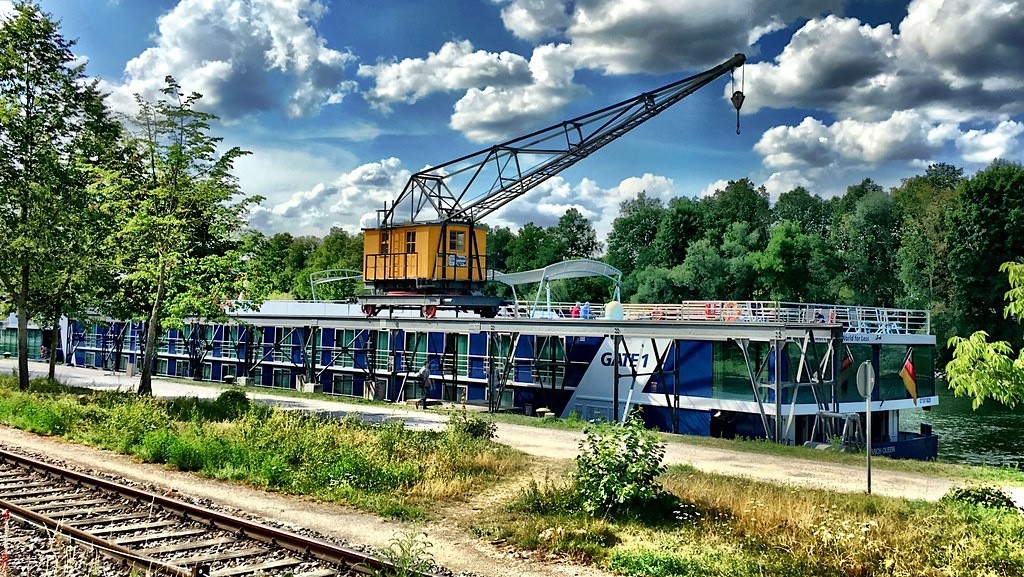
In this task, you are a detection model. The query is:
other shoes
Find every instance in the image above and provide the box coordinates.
[423,407,428,409]
[415,402,419,410]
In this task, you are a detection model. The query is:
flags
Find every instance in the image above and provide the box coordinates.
[897,347,918,407]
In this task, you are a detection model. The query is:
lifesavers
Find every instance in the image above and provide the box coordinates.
[723,302,741,321]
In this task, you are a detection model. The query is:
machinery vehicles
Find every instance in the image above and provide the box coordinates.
[356,52,748,318]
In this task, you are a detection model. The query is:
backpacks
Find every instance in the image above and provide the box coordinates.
[417,368,428,389]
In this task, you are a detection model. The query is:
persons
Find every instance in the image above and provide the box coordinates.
[571,301,593,319]
[814,312,825,324]
[415,360,433,409]
